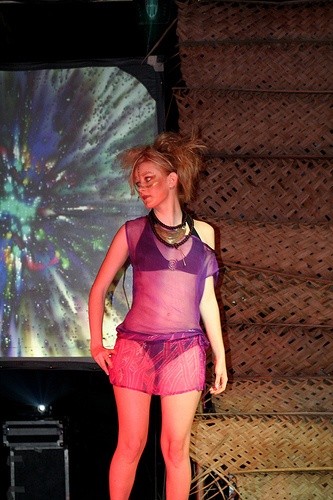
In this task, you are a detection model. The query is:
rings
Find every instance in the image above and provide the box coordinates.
[109,354,112,357]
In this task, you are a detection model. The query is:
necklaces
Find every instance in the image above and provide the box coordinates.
[149,209,194,248]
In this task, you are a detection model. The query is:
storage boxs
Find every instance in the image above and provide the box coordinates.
[1,421,70,500]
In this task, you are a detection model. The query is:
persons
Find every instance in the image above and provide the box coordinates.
[89,124,227,500]
